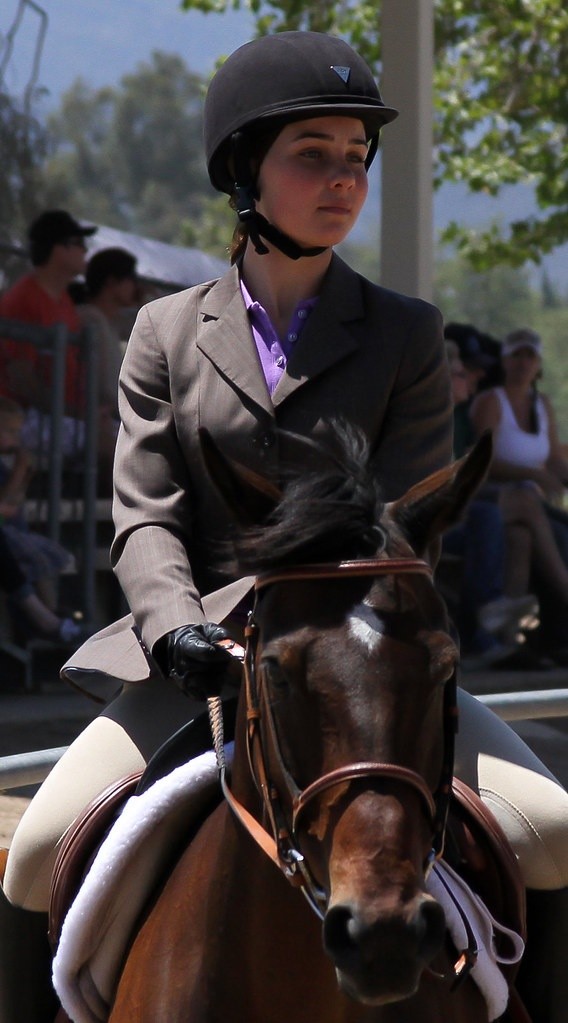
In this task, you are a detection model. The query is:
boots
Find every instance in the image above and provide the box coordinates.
[524,886,568,1023]
[0,886,61,1023]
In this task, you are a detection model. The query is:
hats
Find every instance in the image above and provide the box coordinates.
[30,210,98,244]
[502,329,544,356]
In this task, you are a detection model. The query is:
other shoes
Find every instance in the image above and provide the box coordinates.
[55,618,96,661]
[478,634,555,672]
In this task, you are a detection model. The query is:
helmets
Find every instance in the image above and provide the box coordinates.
[205,30,395,192]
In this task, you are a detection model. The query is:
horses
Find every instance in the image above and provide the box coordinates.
[107,415,530,1023]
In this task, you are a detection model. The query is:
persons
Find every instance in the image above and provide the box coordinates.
[0,33,567,1023]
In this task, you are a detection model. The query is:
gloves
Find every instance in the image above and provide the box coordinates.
[154,622,229,703]
